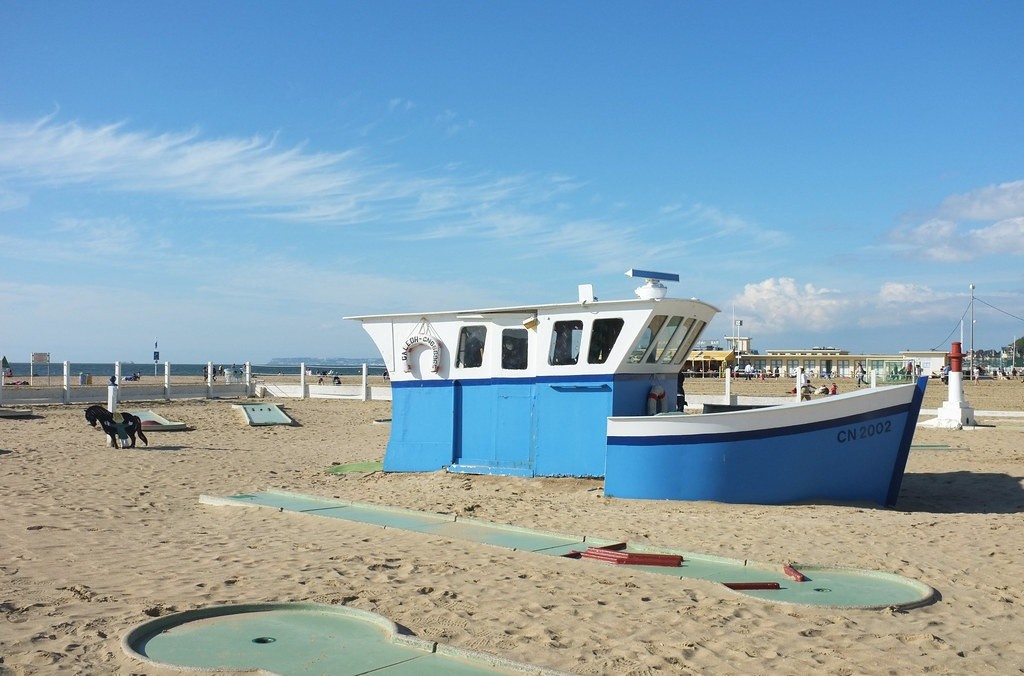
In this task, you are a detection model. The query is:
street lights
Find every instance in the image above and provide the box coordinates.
[735,320,743,367]
[969,283,977,381]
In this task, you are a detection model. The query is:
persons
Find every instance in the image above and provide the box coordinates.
[974,366,980,385]
[382,369,387,383]
[219,363,246,376]
[745,364,773,380]
[734,364,739,378]
[122,371,140,381]
[899,362,912,380]
[916,365,923,376]
[775,367,780,379]
[5,380,29,385]
[306,367,342,386]
[697,367,703,378]
[940,363,951,387]
[893,364,899,380]
[796,366,838,402]
[856,362,869,388]
[203,367,208,381]
[687,367,695,378]
[6,369,13,377]
[213,365,217,381]
[997,368,1022,381]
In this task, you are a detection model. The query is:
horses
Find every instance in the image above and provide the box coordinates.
[83,406,148,449]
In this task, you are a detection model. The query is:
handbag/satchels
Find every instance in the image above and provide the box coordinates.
[863,371,866,374]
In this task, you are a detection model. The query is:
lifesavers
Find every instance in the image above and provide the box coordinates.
[648,385,668,416]
